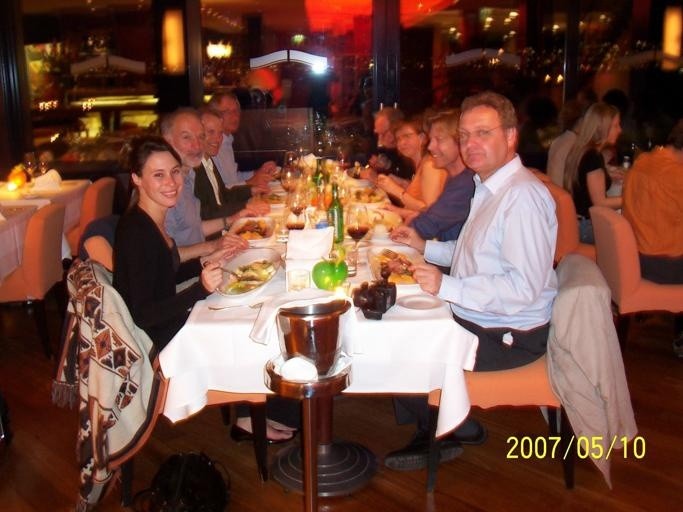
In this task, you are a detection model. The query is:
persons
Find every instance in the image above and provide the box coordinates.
[513,86,683,286]
[383,88,560,468]
[191,106,270,237]
[132,108,257,284]
[112,136,295,444]
[208,92,280,186]
[362,105,479,274]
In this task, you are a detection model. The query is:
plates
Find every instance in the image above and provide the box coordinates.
[366,243,428,289]
[394,292,446,312]
[213,247,280,297]
[225,216,274,244]
[368,209,403,235]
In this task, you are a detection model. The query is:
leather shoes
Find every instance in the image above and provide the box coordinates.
[232,420,294,445]
[384,415,488,472]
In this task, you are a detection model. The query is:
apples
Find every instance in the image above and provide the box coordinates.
[312,261,348,290]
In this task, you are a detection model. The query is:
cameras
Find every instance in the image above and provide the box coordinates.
[350,265,400,321]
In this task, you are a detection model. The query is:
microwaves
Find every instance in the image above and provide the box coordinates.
[326,181,344,243]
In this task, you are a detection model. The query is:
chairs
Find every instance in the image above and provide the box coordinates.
[421,254,610,512]
[64,222,231,429]
[589,204,682,363]
[528,167,550,183]
[67,256,270,511]
[1,203,65,320]
[543,180,598,267]
[62,176,115,260]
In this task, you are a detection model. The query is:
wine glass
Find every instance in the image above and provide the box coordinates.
[21,151,48,184]
[264,149,386,232]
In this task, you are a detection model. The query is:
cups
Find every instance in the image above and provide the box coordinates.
[286,268,310,292]
[330,247,358,275]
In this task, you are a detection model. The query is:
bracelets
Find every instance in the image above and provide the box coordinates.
[222,216,228,231]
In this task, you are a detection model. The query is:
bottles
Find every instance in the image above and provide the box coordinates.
[344,204,368,244]
[620,142,663,173]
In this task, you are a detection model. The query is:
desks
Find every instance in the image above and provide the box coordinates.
[158,164,479,501]
[1,179,93,282]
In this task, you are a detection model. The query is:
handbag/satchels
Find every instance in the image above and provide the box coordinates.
[150,452,230,512]
